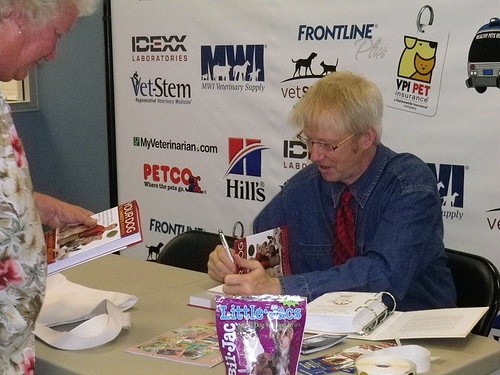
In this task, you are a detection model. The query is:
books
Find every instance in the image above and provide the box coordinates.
[187,226,291,311]
[296,343,394,375]
[125,315,225,368]
[301,290,489,340]
[300,332,347,355]
[40,199,143,275]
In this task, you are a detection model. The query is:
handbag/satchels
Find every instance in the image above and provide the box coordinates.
[33,272,138,351]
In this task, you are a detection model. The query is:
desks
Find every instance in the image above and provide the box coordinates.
[34,254,500,375]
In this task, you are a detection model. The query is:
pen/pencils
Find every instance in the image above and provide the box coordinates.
[217,228,234,264]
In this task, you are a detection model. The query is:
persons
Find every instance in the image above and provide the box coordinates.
[206,72,458,312]
[0,0,98,375]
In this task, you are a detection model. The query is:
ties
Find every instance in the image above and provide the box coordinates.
[332,191,356,267]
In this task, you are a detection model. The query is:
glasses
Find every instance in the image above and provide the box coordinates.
[296,130,356,154]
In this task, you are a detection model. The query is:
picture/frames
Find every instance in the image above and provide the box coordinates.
[0,63,39,115]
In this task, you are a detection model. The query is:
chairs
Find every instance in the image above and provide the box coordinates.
[439,248,500,338]
[156,232,239,275]
